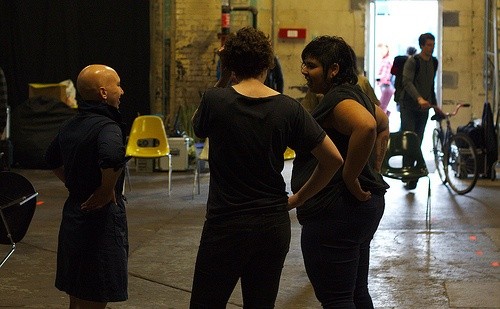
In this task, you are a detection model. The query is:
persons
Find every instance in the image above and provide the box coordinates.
[376,42,396,116]
[390,47,417,111]
[189,26,344,309]
[400,33,446,170]
[291,35,389,309]
[300,74,382,115]
[48,64,129,309]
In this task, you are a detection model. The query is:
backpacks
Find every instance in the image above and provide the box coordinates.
[455,121,485,175]
[394,54,438,102]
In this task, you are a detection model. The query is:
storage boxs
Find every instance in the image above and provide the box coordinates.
[157,138,189,171]
[29,84,66,103]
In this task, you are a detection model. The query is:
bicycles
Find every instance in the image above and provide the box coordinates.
[419,103,482,195]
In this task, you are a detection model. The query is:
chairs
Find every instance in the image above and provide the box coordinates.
[192,137,209,199]
[381,131,431,197]
[124,115,172,197]
